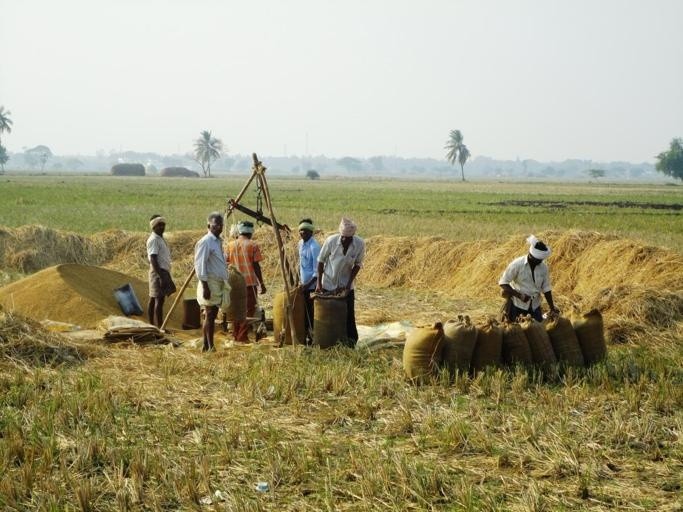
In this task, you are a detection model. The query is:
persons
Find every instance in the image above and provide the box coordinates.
[315,217,367,349]
[295,216,323,345]
[498,240,560,322]
[193,212,230,354]
[145,214,177,334]
[223,221,267,345]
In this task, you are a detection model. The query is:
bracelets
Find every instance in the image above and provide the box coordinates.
[260,281,264,285]
